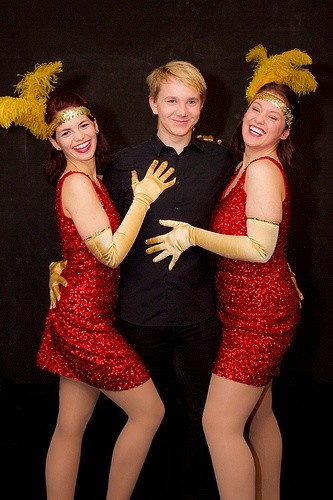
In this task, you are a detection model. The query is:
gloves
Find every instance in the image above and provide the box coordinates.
[286,262,303,309]
[48,259,67,310]
[144,217,278,270]
[82,159,175,269]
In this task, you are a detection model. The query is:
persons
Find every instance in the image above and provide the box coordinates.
[34,89,224,500]
[50,62,304,500]
[145,81,305,500]
